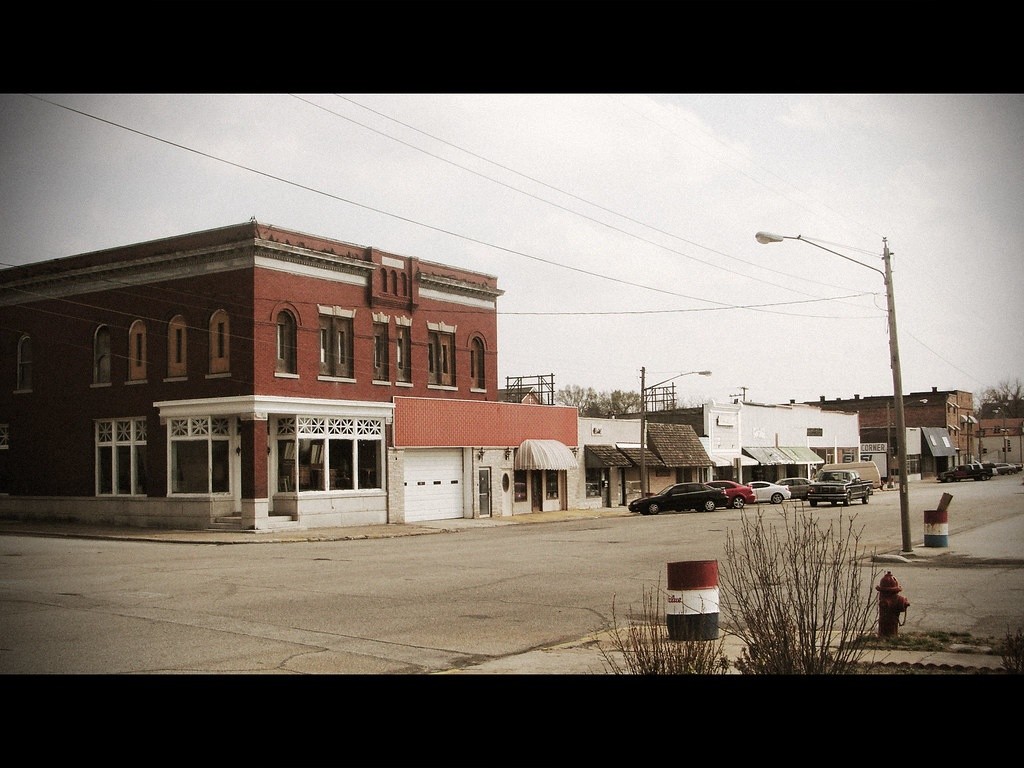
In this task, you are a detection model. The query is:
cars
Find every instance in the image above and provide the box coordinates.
[959,460,1024,478]
[744,480,792,504]
[702,479,755,509]
[628,481,729,515]
[774,477,815,502]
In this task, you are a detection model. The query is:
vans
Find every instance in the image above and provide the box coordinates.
[812,460,882,490]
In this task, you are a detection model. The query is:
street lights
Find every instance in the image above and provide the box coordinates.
[756,231,912,552]
[887,399,928,488]
[992,409,1008,464]
[641,367,712,495]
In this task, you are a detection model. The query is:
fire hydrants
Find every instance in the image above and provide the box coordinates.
[876,569,912,638]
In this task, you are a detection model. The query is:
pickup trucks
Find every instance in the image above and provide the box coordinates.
[808,470,874,508]
[937,465,990,483]
